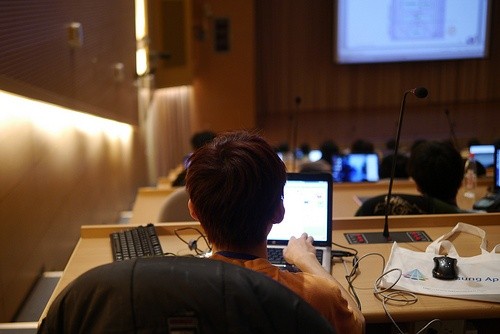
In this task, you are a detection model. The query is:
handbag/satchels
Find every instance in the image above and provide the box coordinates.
[379,221,500,304]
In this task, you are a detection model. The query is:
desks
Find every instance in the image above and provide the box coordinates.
[35,147,500,334]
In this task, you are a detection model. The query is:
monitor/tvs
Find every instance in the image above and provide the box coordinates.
[276,150,380,184]
[467,144,499,188]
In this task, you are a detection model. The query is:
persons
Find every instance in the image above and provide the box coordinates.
[276,137,486,179]
[411,140,485,213]
[186,131,365,334]
[173,131,216,188]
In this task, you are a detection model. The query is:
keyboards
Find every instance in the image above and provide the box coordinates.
[109,223,164,262]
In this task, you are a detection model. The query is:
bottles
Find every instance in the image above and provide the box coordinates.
[462,153,477,199]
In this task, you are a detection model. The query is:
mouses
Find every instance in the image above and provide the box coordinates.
[433,256,457,279]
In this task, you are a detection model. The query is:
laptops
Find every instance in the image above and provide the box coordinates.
[266,172,335,275]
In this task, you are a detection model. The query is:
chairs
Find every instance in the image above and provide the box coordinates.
[36,256,337,334]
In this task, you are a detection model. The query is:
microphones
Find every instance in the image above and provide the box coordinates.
[292,97,302,172]
[383,87,428,235]
